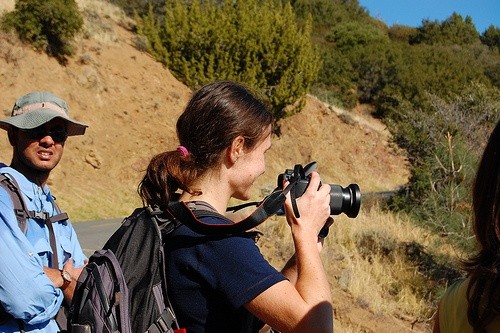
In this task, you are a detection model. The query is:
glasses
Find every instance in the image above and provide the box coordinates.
[18,128,68,143]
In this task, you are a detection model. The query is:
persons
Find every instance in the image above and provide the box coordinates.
[433,120,500,333]
[0,91,92,333]
[153,79,334,333]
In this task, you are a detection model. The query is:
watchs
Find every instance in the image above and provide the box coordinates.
[57,267,73,292]
[318,225,330,238]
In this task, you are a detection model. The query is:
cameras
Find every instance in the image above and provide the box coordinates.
[274,161,361,238]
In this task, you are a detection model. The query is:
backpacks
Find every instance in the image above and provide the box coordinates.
[69,203,236,333]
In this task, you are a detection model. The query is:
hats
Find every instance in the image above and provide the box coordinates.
[0,93,88,136]
